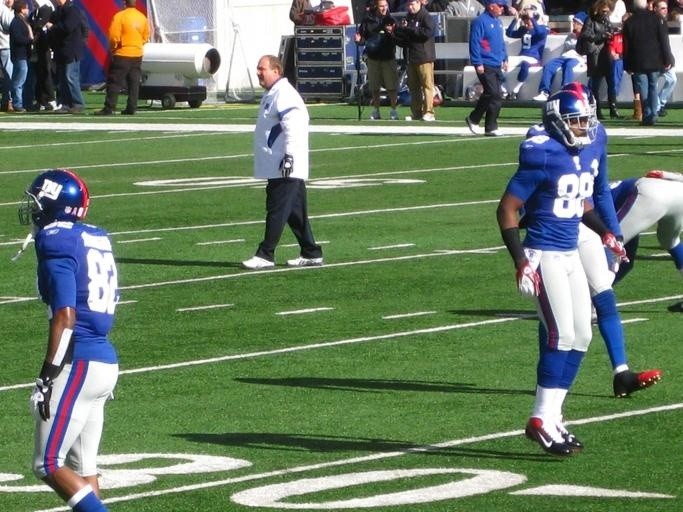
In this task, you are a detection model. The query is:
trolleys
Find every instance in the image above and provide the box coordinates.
[138,42,221,108]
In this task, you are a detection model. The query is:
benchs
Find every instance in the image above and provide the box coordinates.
[460,32,683,107]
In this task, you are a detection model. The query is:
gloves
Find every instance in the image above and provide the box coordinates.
[605,234,631,263]
[516,262,541,296]
[29,375,53,421]
[278,154,293,178]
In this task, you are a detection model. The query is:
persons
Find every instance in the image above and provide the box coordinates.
[495,89,631,457]
[241,53,323,269]
[0,0,92,114]
[385,0,438,120]
[93,0,152,115]
[353,1,548,105]
[532,0,683,126]
[525,81,666,396]
[354,0,402,120]
[464,0,510,136]
[289,0,336,25]
[14,168,121,511]
[605,168,683,311]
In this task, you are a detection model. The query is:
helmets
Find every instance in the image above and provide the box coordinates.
[18,170,90,224]
[521,5,540,20]
[543,91,600,145]
[563,81,596,109]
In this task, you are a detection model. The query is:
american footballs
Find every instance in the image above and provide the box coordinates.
[433,96,440,106]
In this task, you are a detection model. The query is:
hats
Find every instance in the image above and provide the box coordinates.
[487,0,507,6]
[573,12,588,24]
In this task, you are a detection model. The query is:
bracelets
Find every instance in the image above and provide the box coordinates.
[581,210,612,238]
[502,227,528,268]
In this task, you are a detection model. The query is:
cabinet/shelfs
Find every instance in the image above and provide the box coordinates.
[293,24,366,103]
[391,10,515,99]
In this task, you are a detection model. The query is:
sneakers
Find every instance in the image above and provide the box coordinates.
[421,112,435,120]
[56,106,68,113]
[613,370,661,398]
[94,109,113,114]
[526,418,572,456]
[122,109,136,115]
[485,128,502,135]
[640,115,656,126]
[405,113,416,120]
[659,109,666,116]
[465,116,480,135]
[390,110,396,119]
[533,93,549,101]
[68,104,82,112]
[508,92,519,100]
[555,420,584,449]
[242,256,275,269]
[287,256,323,266]
[370,111,380,120]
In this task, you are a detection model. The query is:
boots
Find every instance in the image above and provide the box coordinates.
[610,102,624,118]
[633,100,642,119]
